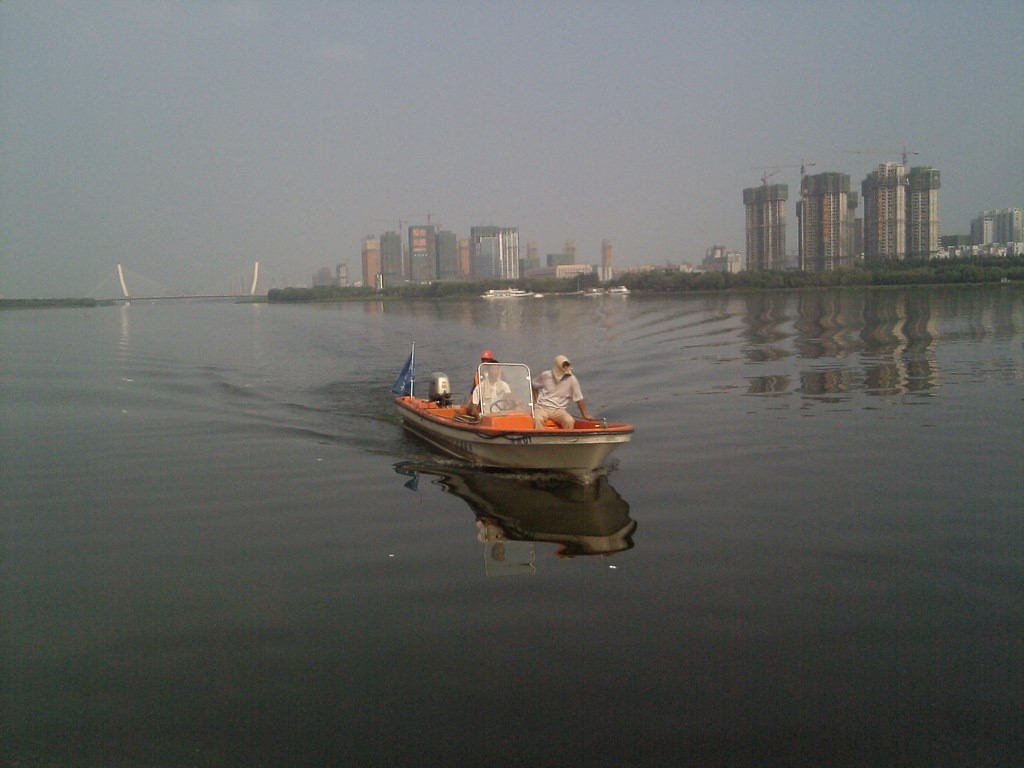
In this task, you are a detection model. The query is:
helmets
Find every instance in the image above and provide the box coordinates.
[480,350,494,359]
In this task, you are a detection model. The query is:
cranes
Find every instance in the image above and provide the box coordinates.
[857,145,919,173]
[434,219,442,273]
[410,211,436,277]
[752,158,815,196]
[760,169,779,184]
[371,217,408,276]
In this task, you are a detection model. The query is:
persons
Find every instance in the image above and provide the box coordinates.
[470,361,532,422]
[467,351,511,409]
[528,355,595,430]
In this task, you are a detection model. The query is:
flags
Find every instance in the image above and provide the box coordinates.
[391,346,416,396]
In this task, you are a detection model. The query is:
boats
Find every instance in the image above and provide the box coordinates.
[481,289,535,300]
[610,286,628,294]
[392,458,637,557]
[395,363,635,475]
